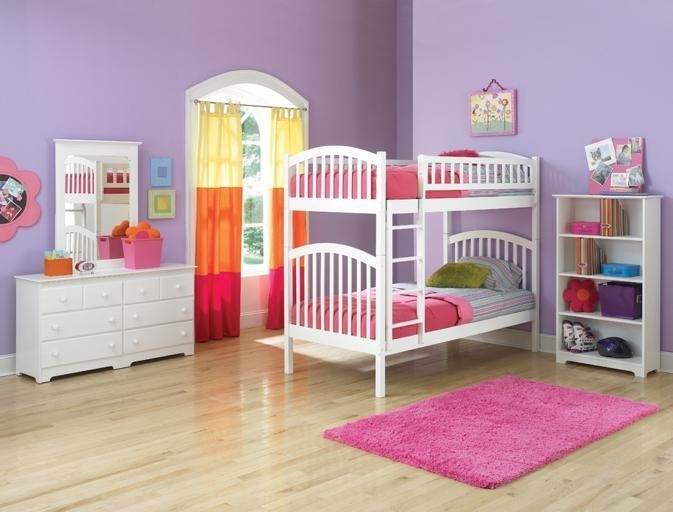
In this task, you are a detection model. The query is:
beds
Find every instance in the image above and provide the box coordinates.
[64,154,129,263]
[283,146,539,398]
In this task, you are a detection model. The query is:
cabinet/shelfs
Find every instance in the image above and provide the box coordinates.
[123,267,196,365]
[553,195,662,375]
[13,275,123,384]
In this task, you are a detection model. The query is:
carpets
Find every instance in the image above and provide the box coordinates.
[323,375,662,489]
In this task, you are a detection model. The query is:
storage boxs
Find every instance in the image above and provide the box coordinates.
[121,237,163,269]
[598,282,642,320]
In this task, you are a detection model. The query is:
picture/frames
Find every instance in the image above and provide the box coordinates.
[148,189,177,218]
[469,89,518,138]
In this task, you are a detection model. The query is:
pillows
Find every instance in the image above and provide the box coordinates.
[424,262,492,288]
[457,256,523,294]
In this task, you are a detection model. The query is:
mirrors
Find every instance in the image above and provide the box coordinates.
[53,138,138,267]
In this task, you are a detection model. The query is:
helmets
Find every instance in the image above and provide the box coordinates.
[595,336,633,359]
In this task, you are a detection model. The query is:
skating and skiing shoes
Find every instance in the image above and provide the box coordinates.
[571,321,597,353]
[561,320,575,352]
